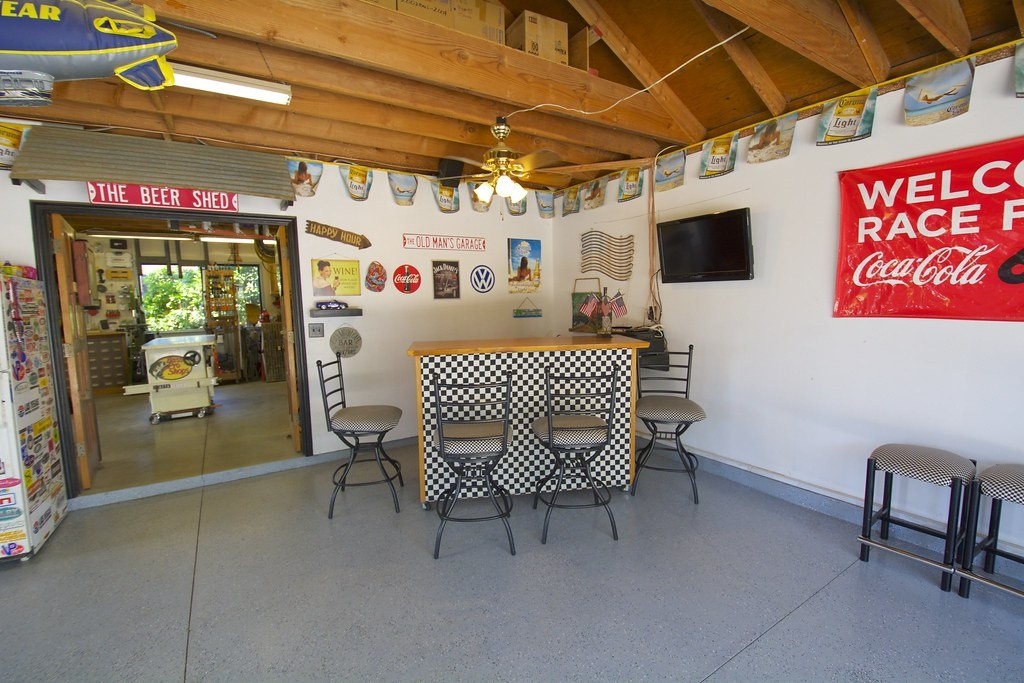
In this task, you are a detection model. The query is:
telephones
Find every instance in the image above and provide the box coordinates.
[638,324,664,331]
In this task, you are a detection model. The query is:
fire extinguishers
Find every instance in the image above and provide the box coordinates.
[261,309,269,323]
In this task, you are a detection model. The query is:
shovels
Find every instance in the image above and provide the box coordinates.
[245,303,259,342]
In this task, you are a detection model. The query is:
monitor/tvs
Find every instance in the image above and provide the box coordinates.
[657,208,754,284]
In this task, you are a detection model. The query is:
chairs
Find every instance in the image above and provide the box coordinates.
[530,364,622,545]
[631,342,705,505]
[430,370,517,559]
[317,352,405,522]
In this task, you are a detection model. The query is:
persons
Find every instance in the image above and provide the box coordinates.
[508,257,533,282]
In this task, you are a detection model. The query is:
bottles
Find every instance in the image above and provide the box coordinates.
[534,260,540,286]
[246,304,260,328]
[404,266,410,292]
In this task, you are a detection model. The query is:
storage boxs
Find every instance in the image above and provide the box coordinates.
[106,252,132,268]
[106,268,132,280]
[397,0,505,50]
[506,9,568,65]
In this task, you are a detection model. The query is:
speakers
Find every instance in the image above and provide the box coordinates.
[437,158,464,187]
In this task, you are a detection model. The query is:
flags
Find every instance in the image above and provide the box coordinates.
[579,292,627,318]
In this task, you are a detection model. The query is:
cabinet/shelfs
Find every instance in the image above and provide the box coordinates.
[205,269,243,386]
[85,329,130,396]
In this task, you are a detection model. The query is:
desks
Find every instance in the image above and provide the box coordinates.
[408,334,650,510]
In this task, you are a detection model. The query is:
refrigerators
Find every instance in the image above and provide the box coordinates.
[0,265,68,563]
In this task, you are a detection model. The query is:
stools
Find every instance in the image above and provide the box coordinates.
[958,465,1024,599]
[857,444,974,592]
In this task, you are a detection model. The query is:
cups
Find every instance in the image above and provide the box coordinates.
[349,166,368,200]
[824,89,870,141]
[623,168,639,198]
[440,184,454,211]
[706,135,732,176]
[566,188,577,212]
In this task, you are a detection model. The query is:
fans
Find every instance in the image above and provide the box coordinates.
[429,117,572,187]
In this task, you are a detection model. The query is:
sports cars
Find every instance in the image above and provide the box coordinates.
[316,298,348,311]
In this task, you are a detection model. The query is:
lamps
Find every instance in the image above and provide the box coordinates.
[166,58,292,106]
[475,172,528,205]
